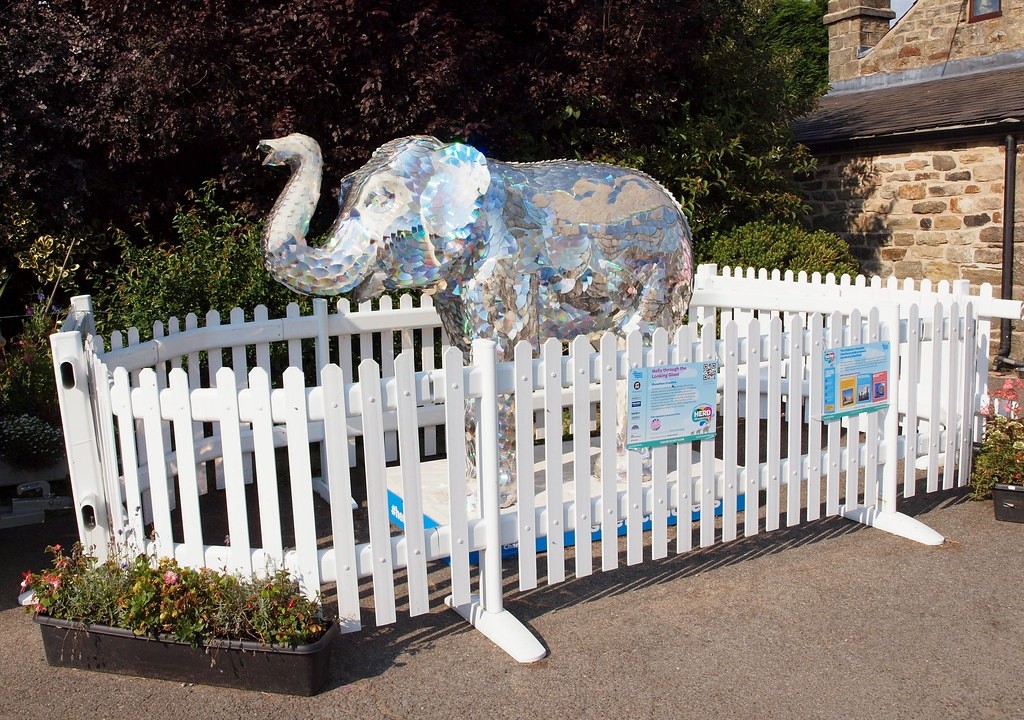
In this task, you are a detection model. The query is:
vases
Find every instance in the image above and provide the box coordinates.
[33,610,339,697]
[991,483,1024,523]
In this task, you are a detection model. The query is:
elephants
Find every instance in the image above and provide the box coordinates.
[255,131,694,510]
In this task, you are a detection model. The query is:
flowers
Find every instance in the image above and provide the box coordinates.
[18,505,337,666]
[964,373,1024,501]
